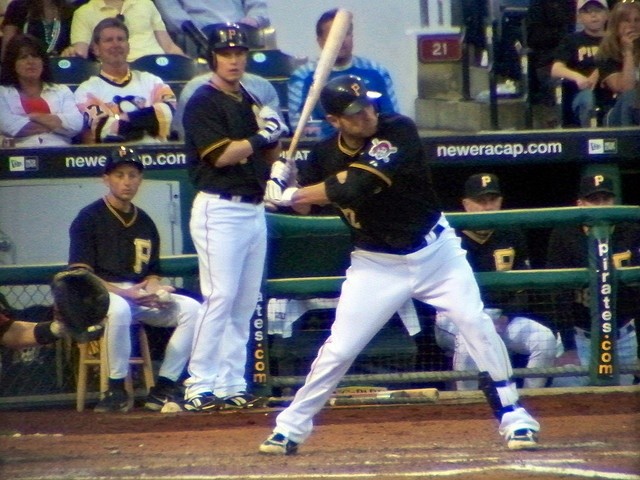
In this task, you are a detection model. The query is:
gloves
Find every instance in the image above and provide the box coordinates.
[258,119,290,144]
[268,156,301,188]
[264,178,298,206]
[251,103,280,129]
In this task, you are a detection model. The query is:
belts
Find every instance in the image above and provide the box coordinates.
[573,325,636,341]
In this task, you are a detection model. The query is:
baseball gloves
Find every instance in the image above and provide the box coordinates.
[52,268,109,344]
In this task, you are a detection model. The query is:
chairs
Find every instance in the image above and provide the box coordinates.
[131,54,197,102]
[241,48,304,132]
[41,55,101,93]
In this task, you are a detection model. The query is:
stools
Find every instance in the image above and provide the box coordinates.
[73,317,156,413]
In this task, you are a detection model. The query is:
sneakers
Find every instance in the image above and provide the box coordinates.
[259,432,296,456]
[221,392,267,410]
[94,389,130,414]
[145,386,183,410]
[508,429,540,449]
[181,392,224,412]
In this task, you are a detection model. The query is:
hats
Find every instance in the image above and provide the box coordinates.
[579,174,617,197]
[577,0,608,12]
[465,174,502,200]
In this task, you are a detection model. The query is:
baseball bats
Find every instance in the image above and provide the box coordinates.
[182,20,288,137]
[264,8,352,212]
[267,388,439,406]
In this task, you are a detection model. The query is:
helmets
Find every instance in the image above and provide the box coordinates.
[207,22,250,69]
[321,74,383,117]
[105,146,145,171]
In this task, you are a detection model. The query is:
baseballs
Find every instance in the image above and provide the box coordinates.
[156,290,170,302]
[163,402,180,412]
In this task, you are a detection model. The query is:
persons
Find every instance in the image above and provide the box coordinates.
[0,33,84,149]
[591,0,640,129]
[549,0,622,129]
[66,144,203,413]
[182,22,289,414]
[1,269,111,352]
[434,172,556,391]
[171,72,287,143]
[71,0,193,62]
[260,74,542,455]
[155,0,271,58]
[545,173,640,388]
[74,18,177,144]
[287,8,399,138]
[0,0,87,59]
